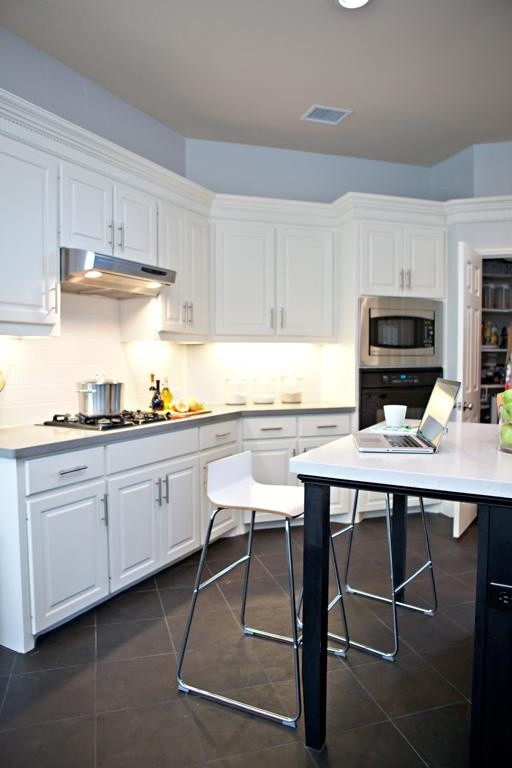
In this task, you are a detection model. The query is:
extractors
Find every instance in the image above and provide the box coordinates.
[59,247,176,303]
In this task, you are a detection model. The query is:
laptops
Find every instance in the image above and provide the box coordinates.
[352,377,461,453]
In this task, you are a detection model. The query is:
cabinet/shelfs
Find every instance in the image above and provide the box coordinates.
[239,398,359,531]
[0,88,58,326]
[60,118,156,267]
[214,193,336,341]
[110,411,201,599]
[0,425,106,657]
[358,195,445,345]
[160,170,214,338]
[198,405,240,542]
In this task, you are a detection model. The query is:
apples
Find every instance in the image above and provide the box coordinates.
[500,404,512,423]
[502,390,512,400]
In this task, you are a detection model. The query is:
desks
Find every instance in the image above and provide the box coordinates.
[289,422,512,768]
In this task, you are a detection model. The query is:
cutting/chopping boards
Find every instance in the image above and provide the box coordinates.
[153,409,212,419]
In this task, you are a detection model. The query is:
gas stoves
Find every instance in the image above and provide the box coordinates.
[43,409,174,432]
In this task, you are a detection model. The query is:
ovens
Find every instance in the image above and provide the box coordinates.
[352,366,451,436]
[357,295,444,368]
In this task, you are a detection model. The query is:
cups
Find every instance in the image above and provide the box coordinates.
[383,404,407,427]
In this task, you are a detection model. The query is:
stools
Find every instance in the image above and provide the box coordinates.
[175,449,349,728]
[297,476,439,664]
